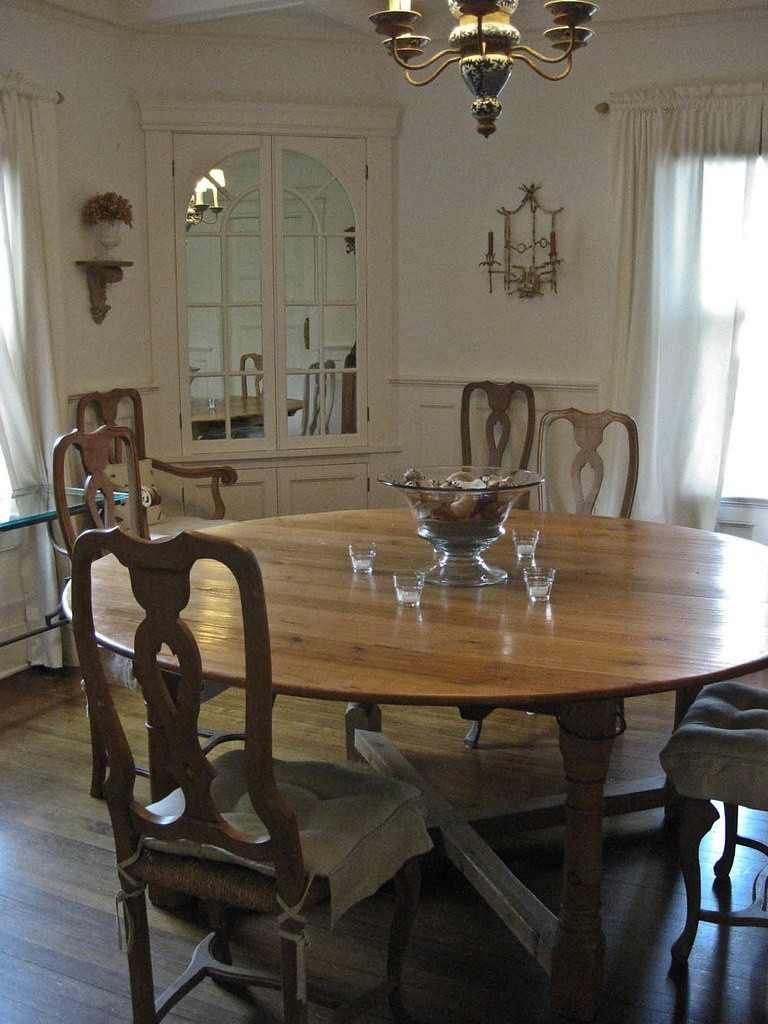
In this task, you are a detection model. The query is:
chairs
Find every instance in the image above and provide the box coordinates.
[239,353,263,397]
[70,521,435,1024]
[50,424,245,801]
[301,359,336,436]
[658,681,768,981]
[460,380,535,511]
[461,407,639,752]
[75,386,239,540]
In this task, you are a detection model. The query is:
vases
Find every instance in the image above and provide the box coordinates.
[92,219,125,260]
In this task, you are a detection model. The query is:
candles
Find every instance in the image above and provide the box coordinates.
[488,231,493,255]
[550,231,556,253]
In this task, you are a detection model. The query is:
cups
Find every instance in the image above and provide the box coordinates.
[512,528,540,560]
[207,398,217,408]
[393,569,425,608]
[523,564,555,603]
[349,540,375,574]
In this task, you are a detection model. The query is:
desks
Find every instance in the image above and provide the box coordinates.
[189,396,305,440]
[0,482,130,679]
[56,504,768,1024]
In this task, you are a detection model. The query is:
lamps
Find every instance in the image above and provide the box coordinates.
[185,168,226,245]
[366,0,603,141]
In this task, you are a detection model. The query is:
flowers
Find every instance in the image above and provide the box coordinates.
[80,190,135,231]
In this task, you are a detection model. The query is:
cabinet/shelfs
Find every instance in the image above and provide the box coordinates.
[341,340,357,435]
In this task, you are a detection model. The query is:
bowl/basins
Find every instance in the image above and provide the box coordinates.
[377,464,544,588]
